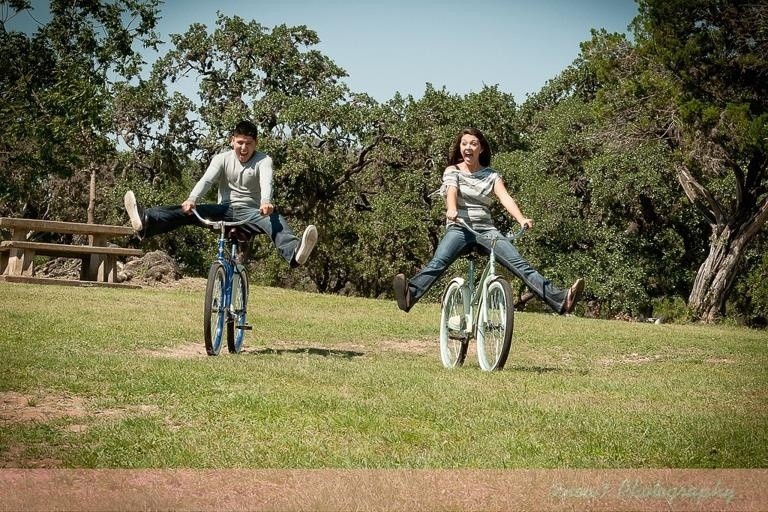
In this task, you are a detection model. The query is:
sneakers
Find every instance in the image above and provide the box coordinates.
[294,223,320,266]
[124,190,146,233]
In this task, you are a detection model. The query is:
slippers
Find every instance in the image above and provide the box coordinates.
[566,278,585,314]
[393,272,412,312]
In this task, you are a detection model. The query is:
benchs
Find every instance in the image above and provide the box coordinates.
[0,240,142,289]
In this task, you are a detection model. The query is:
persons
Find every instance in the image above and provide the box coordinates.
[123,120,318,270]
[392,126,586,315]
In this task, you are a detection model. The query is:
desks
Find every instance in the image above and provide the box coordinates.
[0,216,134,282]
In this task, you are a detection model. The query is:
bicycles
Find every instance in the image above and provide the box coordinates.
[435,211,528,376]
[184,206,268,357]
